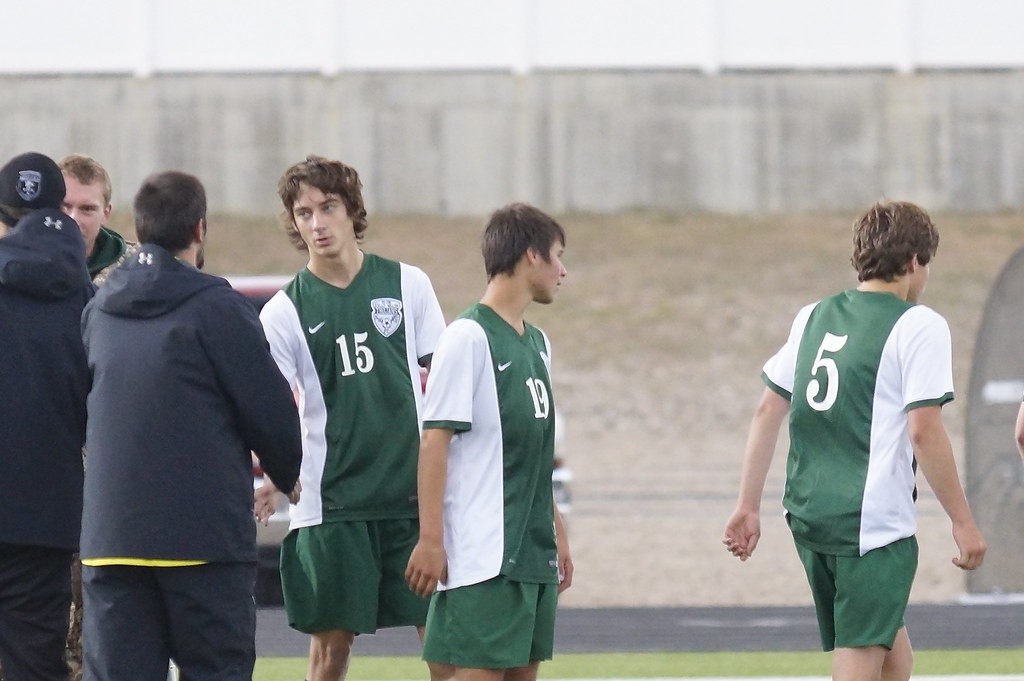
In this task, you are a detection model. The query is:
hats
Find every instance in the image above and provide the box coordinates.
[0,152,66,227]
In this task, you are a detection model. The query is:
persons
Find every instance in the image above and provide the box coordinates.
[720,202,987,680]
[254,156,447,681]
[0,146,140,681]
[403,202,573,681]
[75,172,304,681]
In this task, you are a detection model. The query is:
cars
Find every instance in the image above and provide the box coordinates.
[229,275,572,574]
